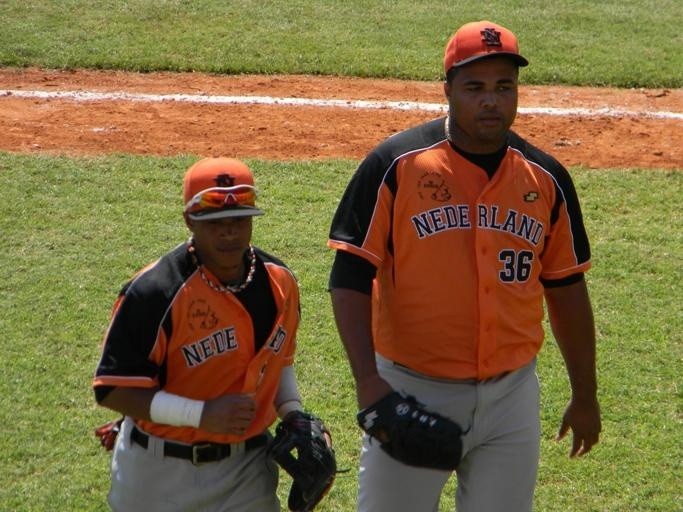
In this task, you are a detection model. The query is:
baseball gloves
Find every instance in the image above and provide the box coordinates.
[356,393,462,470]
[267,410,350,511]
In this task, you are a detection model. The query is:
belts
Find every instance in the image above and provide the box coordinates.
[128,426,268,461]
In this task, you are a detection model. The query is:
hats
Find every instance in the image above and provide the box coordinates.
[444,21,528,78]
[185,157,264,220]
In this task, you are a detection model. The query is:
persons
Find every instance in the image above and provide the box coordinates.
[93,156,338,512]
[321,17,606,512]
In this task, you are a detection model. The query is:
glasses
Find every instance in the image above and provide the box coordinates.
[185,184,255,210]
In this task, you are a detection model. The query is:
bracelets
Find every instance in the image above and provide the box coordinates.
[147,390,206,430]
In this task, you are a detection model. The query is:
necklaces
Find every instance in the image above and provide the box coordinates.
[185,237,258,294]
